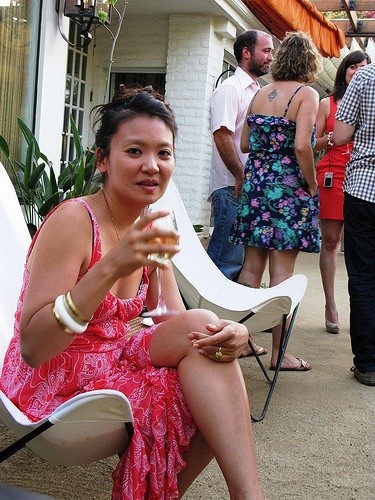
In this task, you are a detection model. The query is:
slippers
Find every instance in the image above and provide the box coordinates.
[238,347,266,358]
[270,357,312,371]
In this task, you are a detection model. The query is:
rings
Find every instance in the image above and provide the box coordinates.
[215,346,222,359]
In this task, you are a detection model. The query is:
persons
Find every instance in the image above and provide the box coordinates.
[333,63,375,386]
[314,50,371,332]
[0,86,264,500]
[229,30,319,371]
[207,29,274,280]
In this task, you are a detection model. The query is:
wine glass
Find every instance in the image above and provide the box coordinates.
[142,206,182,317]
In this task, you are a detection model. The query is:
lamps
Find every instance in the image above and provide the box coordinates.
[56,0,112,47]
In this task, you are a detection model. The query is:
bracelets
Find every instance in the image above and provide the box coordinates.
[52,291,94,335]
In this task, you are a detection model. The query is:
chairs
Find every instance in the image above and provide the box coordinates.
[151,177,306,422]
[0,162,135,468]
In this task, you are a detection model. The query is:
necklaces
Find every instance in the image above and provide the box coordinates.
[101,189,121,240]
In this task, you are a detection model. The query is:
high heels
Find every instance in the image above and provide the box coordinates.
[324,308,341,333]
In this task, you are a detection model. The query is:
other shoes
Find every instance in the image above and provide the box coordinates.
[349,365,375,386]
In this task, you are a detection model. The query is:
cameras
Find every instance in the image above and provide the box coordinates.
[323,172,333,187]
